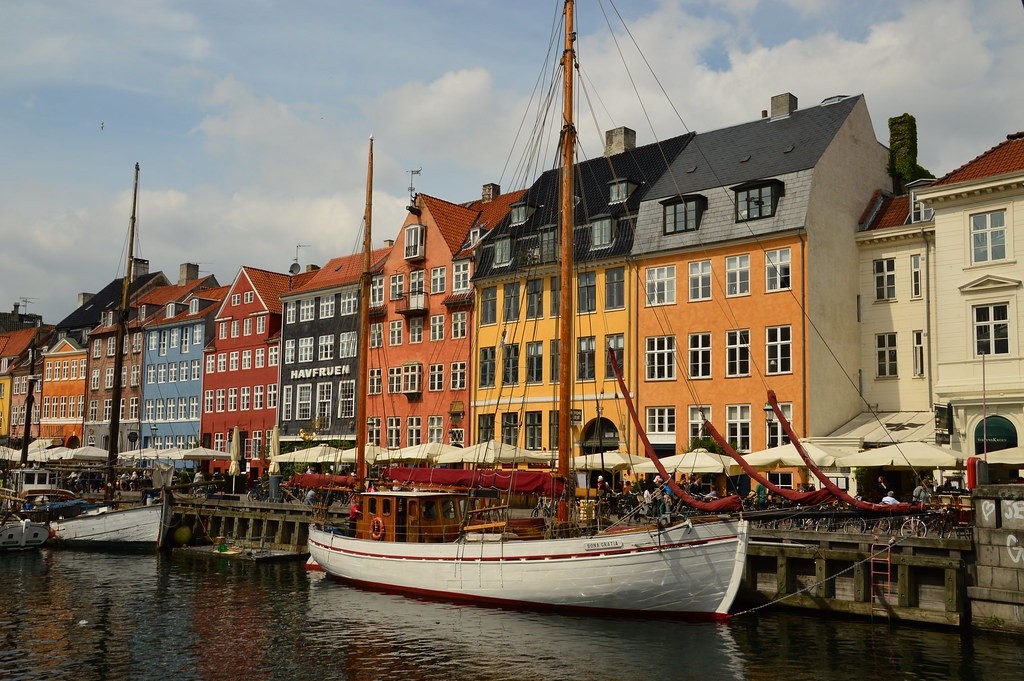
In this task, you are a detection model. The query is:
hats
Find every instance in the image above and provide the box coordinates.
[923,479,929,486]
[887,491,894,496]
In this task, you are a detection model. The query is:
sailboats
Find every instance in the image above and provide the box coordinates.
[0,161,174,554]
[278,0,946,622]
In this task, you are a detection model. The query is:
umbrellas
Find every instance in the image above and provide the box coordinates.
[963,446,1024,470]
[729,442,835,494]
[0,445,231,463]
[268,425,280,476]
[547,451,652,492]
[834,442,969,486]
[272,439,553,470]
[631,448,734,475]
[228,425,241,494]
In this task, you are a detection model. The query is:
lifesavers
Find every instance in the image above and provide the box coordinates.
[370,517,384,539]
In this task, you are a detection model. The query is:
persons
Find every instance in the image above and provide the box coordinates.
[754,480,808,509]
[69,466,152,494]
[877,474,930,505]
[297,464,420,505]
[174,465,205,494]
[349,500,363,537]
[597,475,718,522]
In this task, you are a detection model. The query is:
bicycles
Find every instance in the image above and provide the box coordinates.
[532,497,557,517]
[751,504,972,539]
[247,489,259,501]
[188,487,208,504]
